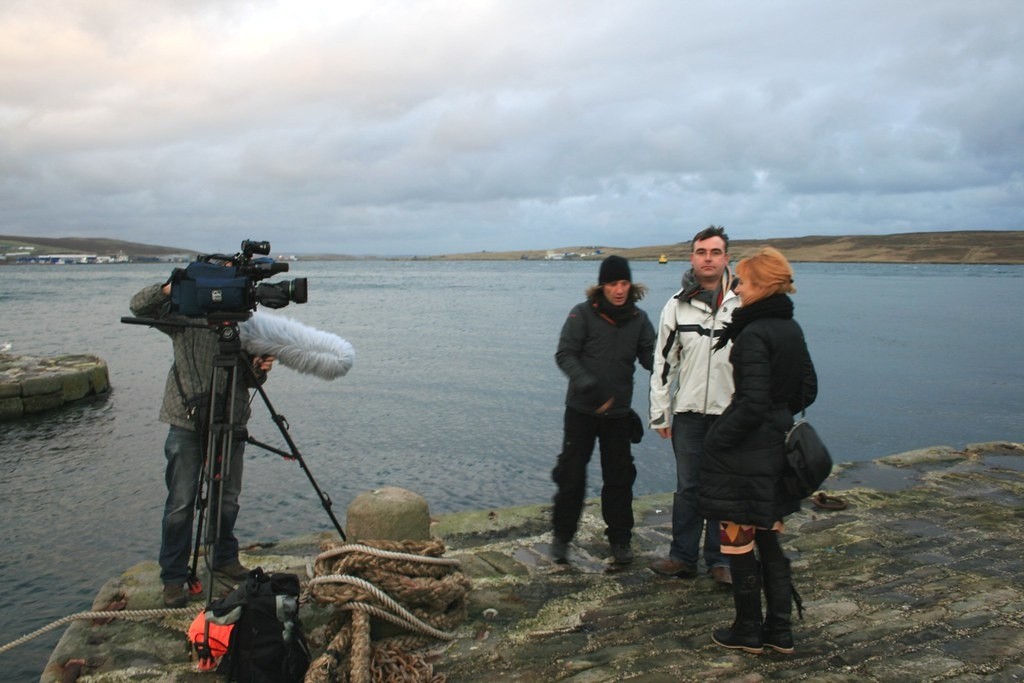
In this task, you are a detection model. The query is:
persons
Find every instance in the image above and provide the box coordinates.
[692,246,818,652]
[131,256,275,606]
[648,227,742,583]
[548,255,657,565]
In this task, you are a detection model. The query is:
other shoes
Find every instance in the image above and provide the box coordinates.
[163,580,187,608]
[550,535,566,563]
[212,557,250,582]
[611,539,634,564]
[708,562,733,585]
[650,552,698,578]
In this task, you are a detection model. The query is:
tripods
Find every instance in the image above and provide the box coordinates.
[119,311,347,673]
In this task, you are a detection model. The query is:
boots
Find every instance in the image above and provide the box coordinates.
[710,565,765,654]
[762,557,804,654]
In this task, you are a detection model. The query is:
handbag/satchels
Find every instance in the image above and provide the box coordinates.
[784,419,833,500]
[631,408,645,444]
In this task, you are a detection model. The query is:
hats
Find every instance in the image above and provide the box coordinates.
[598,255,631,286]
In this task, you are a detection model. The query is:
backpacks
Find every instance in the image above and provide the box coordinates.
[217,567,310,683]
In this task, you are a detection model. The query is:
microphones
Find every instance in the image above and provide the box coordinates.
[237,311,355,380]
